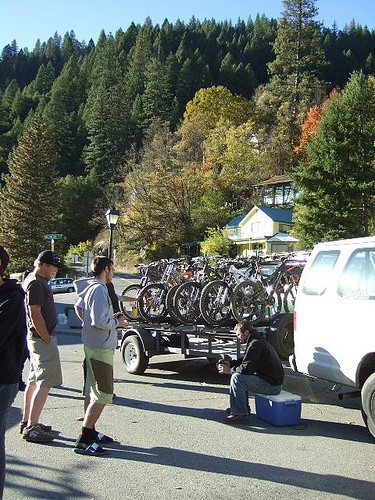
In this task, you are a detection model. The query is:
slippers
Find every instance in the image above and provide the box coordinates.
[74,443,108,455]
[94,432,113,444]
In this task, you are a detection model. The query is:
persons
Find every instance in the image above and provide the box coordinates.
[21,251,62,442]
[217,320,285,424]
[73,256,126,456]
[0,248,27,500]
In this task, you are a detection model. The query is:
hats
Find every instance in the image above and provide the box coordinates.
[37,250,63,268]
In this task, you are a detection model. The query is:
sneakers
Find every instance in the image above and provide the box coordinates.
[22,427,55,443]
[19,421,53,433]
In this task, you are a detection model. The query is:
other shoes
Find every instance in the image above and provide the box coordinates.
[226,407,250,415]
[226,413,249,423]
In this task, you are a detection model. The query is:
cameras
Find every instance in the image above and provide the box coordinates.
[218,359,225,364]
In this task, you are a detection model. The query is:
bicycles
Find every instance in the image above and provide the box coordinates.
[119,251,310,326]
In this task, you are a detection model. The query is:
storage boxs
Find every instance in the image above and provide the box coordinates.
[254,390,302,428]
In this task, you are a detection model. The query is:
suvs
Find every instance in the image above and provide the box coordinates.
[288,235,375,437]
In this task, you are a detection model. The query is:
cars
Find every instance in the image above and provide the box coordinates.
[48,278,75,293]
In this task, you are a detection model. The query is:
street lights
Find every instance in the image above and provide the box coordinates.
[104,209,120,260]
